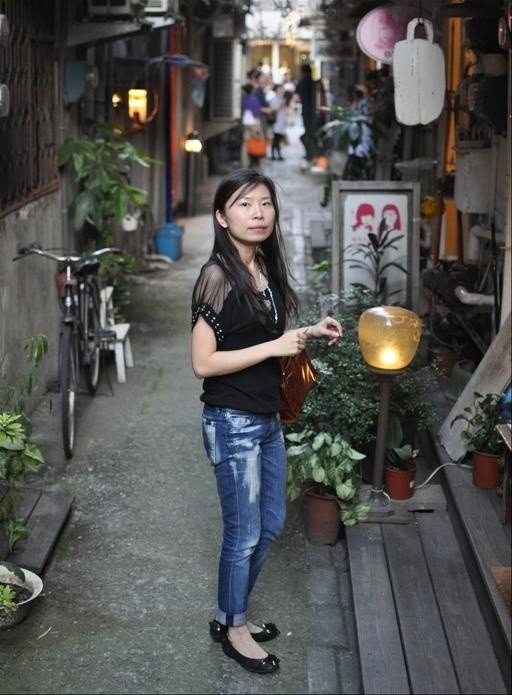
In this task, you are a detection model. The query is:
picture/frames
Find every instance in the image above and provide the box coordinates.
[331,179,421,303]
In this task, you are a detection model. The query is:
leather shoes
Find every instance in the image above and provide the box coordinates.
[210,617,279,641]
[223,635,280,674]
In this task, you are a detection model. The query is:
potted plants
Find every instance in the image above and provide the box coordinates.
[450,392,511,489]
[0,564,43,631]
[286,426,370,547]
[384,416,419,500]
[55,256,79,297]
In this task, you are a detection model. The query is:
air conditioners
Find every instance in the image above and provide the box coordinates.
[89,0,171,15]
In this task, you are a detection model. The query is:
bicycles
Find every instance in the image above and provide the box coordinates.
[16,244,122,463]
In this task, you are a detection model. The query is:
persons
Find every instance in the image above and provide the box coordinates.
[240,62,389,169]
[191,169,343,673]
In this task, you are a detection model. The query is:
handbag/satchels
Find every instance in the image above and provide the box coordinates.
[279,350,318,424]
[247,139,266,157]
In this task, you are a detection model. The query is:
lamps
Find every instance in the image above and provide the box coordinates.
[357,305,421,523]
